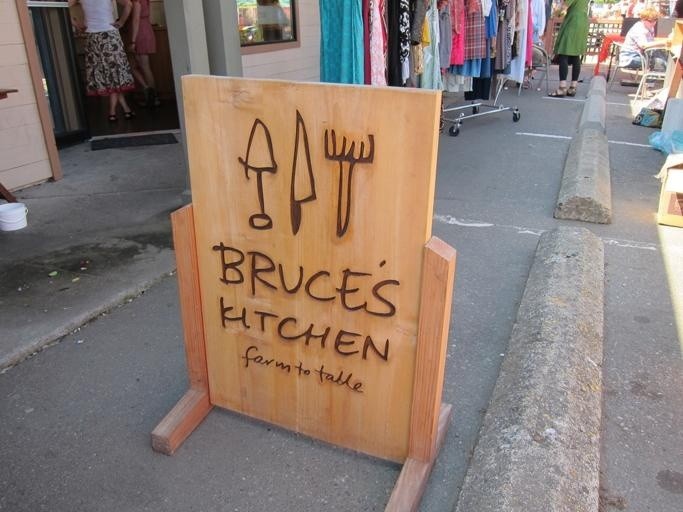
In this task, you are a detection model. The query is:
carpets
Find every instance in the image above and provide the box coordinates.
[88,132,179,151]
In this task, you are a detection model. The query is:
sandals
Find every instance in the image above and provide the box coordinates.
[567,87,576,96]
[548,87,567,98]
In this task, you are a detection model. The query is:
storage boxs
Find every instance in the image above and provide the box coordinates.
[651,153,683,229]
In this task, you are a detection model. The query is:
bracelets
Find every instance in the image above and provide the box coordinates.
[128,41,136,44]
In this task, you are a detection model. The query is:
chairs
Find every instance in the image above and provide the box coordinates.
[517,42,549,94]
[606,41,670,107]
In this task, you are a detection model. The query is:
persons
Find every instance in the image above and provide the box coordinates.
[548,0,590,98]
[619,7,672,88]
[127,0,161,107]
[68,0,138,123]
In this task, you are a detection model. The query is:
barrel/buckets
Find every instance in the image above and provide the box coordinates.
[0,202,29,233]
